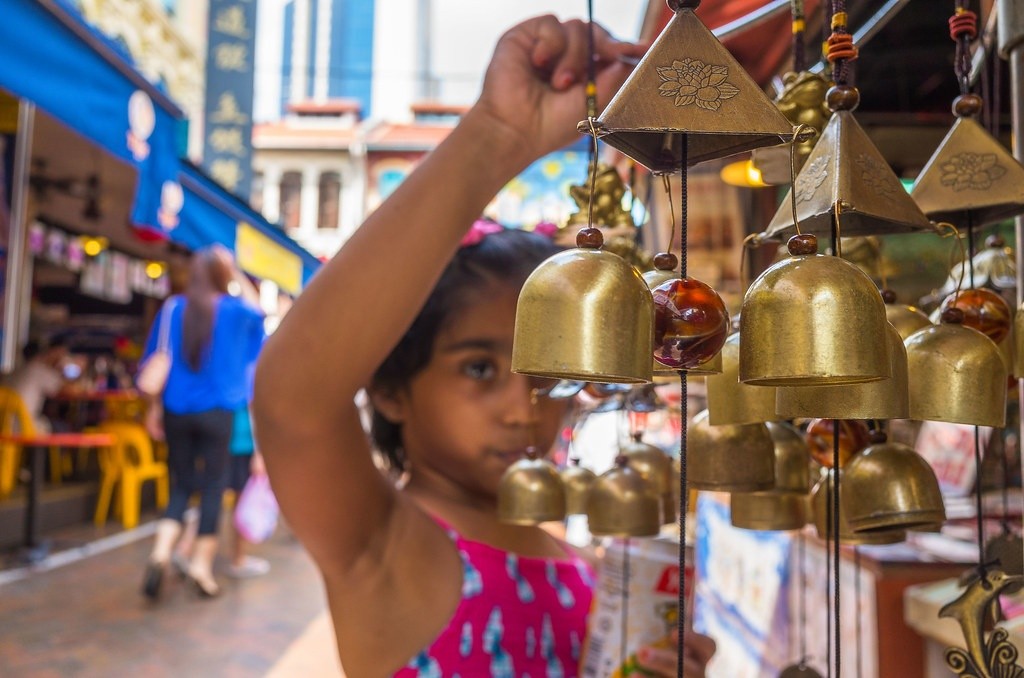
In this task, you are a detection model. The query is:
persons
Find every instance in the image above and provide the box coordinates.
[252,15,716,678]
[133,247,271,603]
[11,338,108,483]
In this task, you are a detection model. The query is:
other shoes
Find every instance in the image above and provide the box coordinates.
[171,549,188,577]
[225,557,268,577]
[139,558,170,603]
[186,563,220,596]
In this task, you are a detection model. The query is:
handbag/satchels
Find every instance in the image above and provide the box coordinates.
[234,475,277,545]
[135,294,178,396]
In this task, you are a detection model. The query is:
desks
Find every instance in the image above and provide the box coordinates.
[1,433,118,562]
[47,389,138,484]
[691,478,1023,678]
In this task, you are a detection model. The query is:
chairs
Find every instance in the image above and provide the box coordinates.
[94,421,169,531]
[0,388,61,495]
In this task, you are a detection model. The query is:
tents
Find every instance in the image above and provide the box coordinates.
[3,0,184,167]
[125,145,320,300]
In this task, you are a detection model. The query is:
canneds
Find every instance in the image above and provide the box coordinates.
[573,533,694,678]
